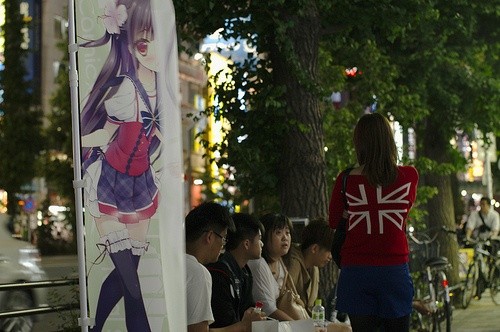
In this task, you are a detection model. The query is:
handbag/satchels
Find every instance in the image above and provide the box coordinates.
[330,167,353,267]
[478,211,492,233]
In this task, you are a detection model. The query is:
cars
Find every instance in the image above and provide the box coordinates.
[0,214,48,332]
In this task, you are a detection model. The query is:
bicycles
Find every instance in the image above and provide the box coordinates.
[405,222,460,332]
[462,235,500,310]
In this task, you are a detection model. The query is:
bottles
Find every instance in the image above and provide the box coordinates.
[253,302,263,313]
[312,299,325,327]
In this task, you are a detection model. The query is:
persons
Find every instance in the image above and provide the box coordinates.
[185,201,266,332]
[206,213,264,329]
[282,216,352,332]
[247,211,327,332]
[459,196,500,297]
[327,112,419,332]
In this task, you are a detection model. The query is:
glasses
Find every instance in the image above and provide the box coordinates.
[201,229,229,245]
